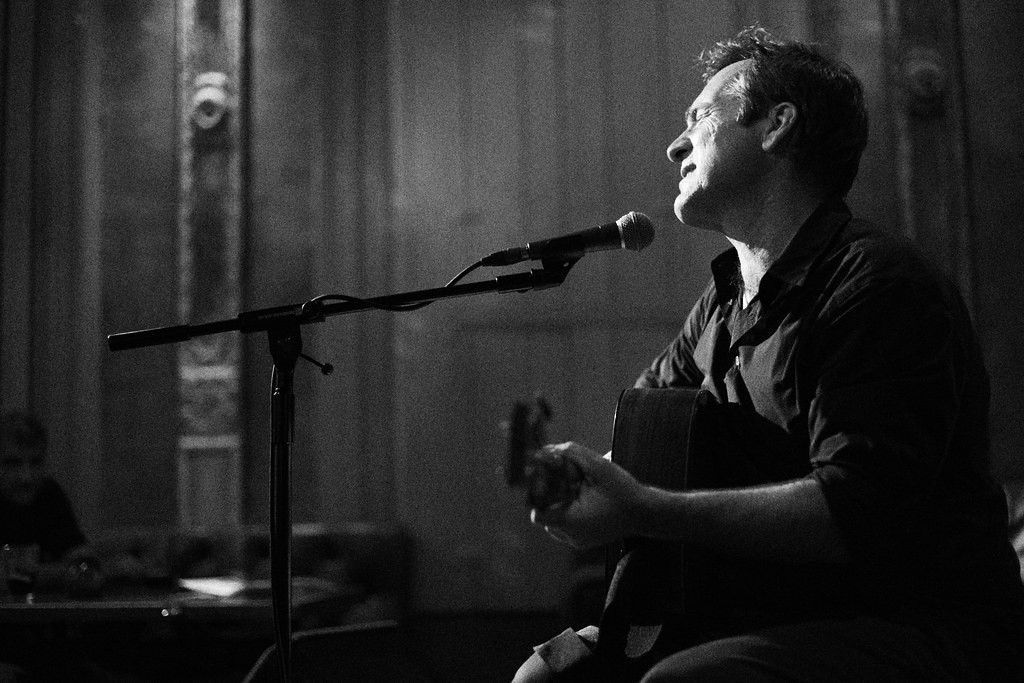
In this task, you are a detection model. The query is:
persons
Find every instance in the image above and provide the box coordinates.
[512,25,1023,682]
[1,411,96,595]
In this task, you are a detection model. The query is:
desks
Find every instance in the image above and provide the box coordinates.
[1,587,343,682]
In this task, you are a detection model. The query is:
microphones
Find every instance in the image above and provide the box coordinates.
[480,211,655,267]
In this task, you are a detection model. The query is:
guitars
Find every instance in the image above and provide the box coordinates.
[502,384,721,626]
